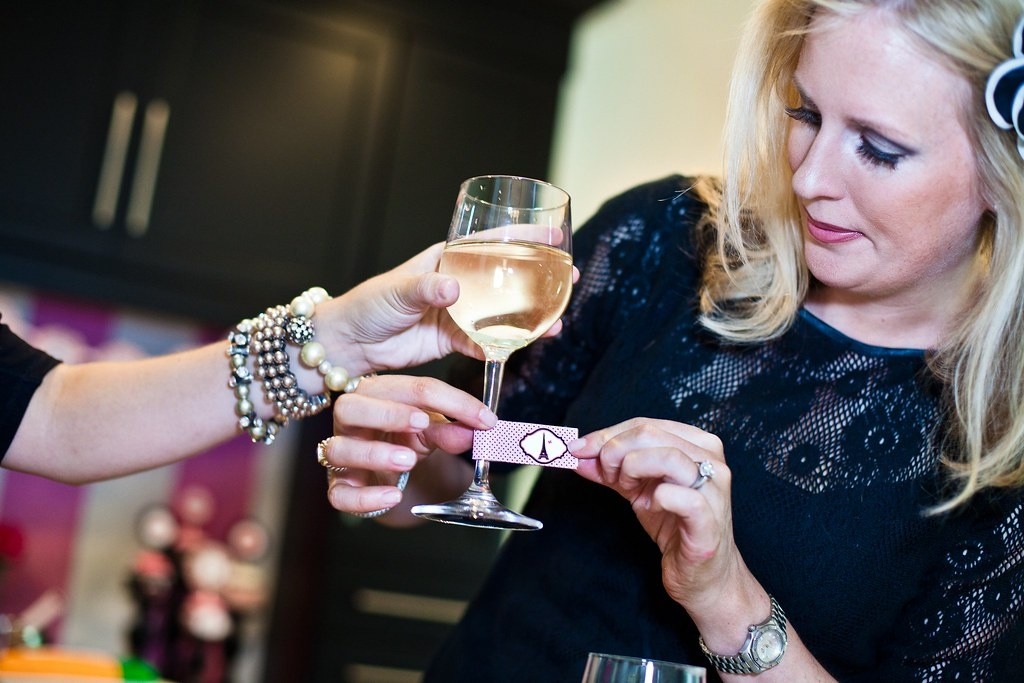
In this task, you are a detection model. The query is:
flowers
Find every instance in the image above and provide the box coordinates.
[984,16,1024,161]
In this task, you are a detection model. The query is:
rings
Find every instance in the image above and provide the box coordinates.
[317,436,348,471]
[691,460,714,489]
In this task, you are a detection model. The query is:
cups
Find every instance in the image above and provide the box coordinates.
[580,651,707,683]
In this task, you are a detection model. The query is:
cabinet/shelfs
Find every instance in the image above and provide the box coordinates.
[0,0,573,326]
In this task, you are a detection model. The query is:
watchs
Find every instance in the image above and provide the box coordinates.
[699,594,787,675]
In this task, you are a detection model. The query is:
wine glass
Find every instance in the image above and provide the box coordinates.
[411,175,572,529]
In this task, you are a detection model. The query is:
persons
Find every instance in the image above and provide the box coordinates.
[0,224,579,484]
[323,0,1024,683]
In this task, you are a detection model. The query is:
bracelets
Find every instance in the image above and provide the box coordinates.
[225,286,363,446]
